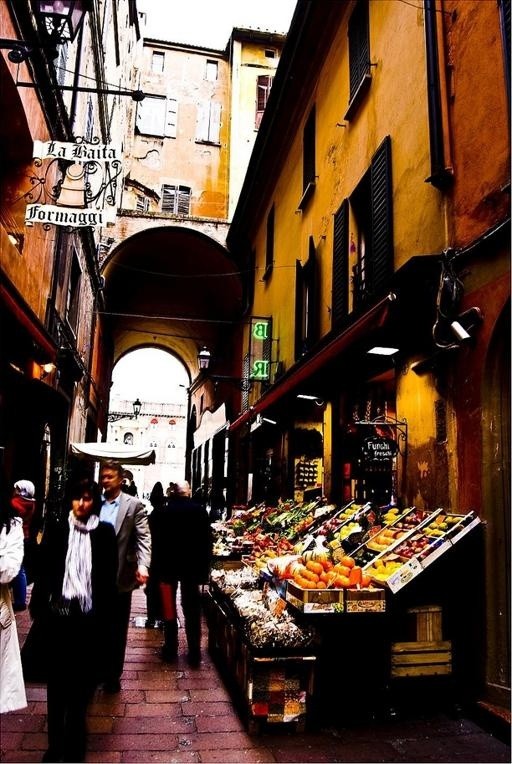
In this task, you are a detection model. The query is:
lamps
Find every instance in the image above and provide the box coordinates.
[197,344,211,371]
[296,379,326,411]
[0,1,93,64]
[108,398,142,424]
[364,329,406,373]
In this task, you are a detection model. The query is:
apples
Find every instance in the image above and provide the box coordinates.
[399,537,431,561]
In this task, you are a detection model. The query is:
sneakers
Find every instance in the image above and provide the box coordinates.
[145,619,165,629]
[104,679,121,693]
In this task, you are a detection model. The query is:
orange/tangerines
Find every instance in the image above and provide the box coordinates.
[295,557,371,588]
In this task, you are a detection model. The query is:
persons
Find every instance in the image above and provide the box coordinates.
[1,461,215,764]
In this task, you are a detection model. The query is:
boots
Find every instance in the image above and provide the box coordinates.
[158,617,179,661]
[185,618,201,664]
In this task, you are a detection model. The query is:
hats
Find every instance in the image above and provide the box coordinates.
[14,478,37,502]
